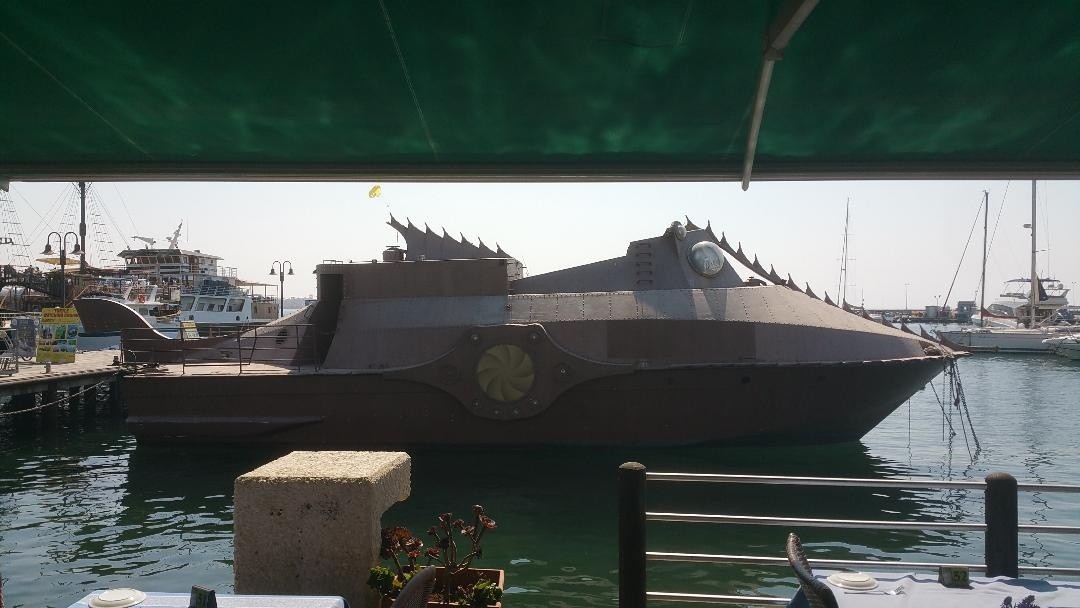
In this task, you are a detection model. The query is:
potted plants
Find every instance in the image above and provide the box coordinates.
[365,501,506,608]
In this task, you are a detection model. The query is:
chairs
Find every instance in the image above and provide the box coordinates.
[785,529,840,608]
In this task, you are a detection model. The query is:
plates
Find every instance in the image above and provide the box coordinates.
[88,588,146,608]
[827,572,879,589]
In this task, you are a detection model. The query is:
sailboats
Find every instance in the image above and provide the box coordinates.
[889,180,1080,360]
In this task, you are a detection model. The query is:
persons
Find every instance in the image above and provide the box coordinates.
[27,265,57,273]
[1053,309,1068,326]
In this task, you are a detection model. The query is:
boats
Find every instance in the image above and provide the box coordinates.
[120,214,973,445]
[69,220,281,337]
[381,562,506,608]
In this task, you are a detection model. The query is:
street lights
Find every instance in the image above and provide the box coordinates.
[270,261,295,316]
[41,232,84,308]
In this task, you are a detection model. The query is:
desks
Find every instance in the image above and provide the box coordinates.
[811,568,1080,608]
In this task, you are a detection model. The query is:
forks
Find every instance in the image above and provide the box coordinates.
[844,585,904,595]
